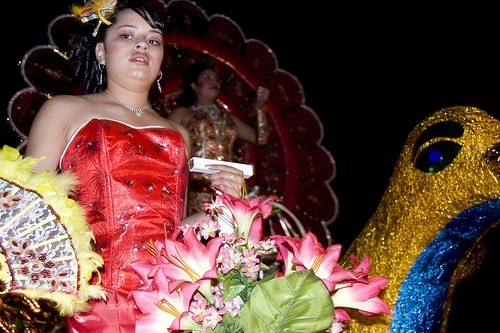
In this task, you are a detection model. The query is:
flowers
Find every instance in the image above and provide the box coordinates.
[132,190,391,333]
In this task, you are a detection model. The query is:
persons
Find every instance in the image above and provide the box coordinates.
[169,65,270,161]
[22,1,243,333]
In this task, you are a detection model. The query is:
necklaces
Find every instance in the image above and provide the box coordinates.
[105,88,152,118]
